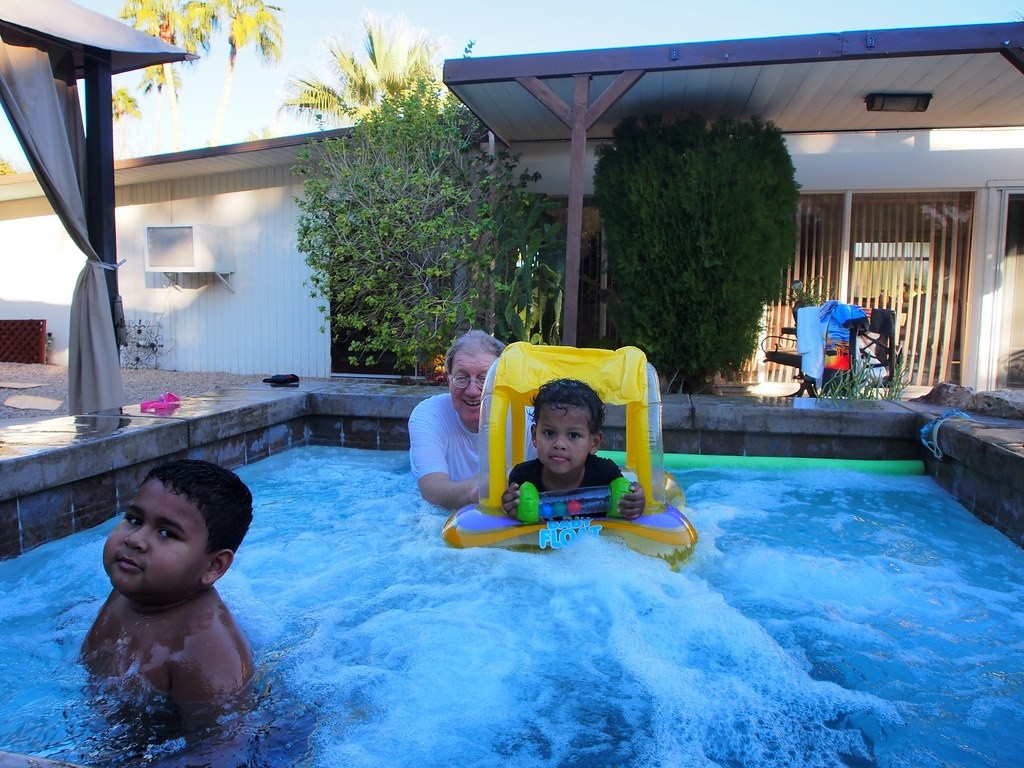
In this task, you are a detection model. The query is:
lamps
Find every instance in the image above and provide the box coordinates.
[864,93,934,113]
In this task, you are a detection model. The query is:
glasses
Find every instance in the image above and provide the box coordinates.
[447,372,486,389]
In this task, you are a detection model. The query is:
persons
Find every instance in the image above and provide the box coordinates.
[407,329,548,512]
[502,379,647,521]
[79,459,257,710]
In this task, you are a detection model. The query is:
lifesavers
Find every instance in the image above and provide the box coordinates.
[440,341,697,573]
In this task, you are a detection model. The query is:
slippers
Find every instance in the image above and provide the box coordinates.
[263,374,299,387]
[141,391,181,415]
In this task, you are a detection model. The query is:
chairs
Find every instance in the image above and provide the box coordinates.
[762,328,818,398]
